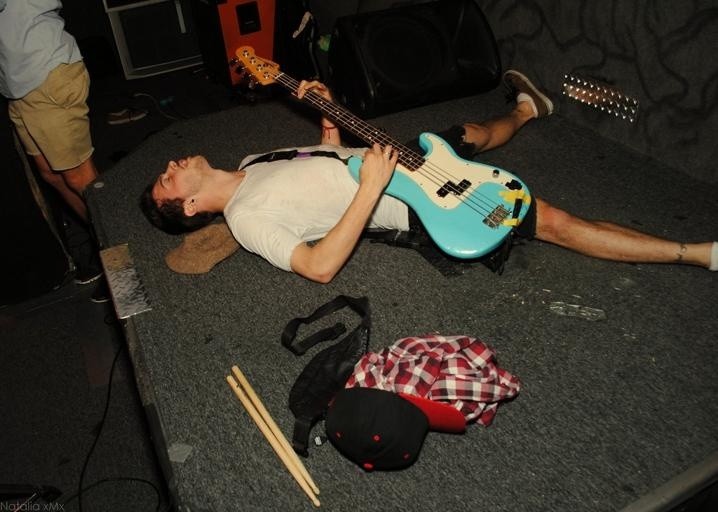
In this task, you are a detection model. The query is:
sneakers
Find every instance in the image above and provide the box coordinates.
[108,108,145,125]
[90,286,110,303]
[503,70,553,119]
[73,269,103,285]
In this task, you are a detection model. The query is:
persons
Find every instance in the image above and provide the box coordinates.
[141,68,718,284]
[0,0,112,303]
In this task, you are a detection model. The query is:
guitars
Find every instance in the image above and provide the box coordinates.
[231,46,532,262]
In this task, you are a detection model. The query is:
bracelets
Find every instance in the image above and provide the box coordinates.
[321,124,339,139]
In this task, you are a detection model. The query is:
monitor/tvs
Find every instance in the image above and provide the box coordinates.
[108,0,204,81]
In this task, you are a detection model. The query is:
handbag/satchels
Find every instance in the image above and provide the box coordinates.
[281,294,372,458]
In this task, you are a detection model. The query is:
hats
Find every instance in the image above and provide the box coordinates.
[165,223,240,275]
[326,386,466,474]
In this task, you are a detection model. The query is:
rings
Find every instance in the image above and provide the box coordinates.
[383,151,392,155]
